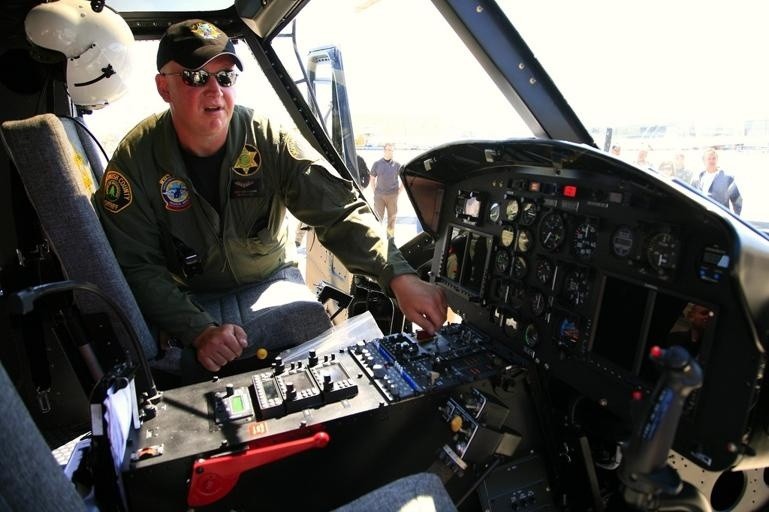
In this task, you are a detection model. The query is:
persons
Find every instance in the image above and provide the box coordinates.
[612,144,621,158]
[690,148,743,216]
[370,143,405,236]
[91,18,448,372]
[633,149,653,168]
[669,152,693,183]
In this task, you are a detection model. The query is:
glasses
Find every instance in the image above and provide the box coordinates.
[161,69,239,87]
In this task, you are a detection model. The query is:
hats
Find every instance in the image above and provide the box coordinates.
[156,19,243,71]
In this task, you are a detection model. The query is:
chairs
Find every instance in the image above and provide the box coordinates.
[0,368,459,512]
[2,112,330,388]
